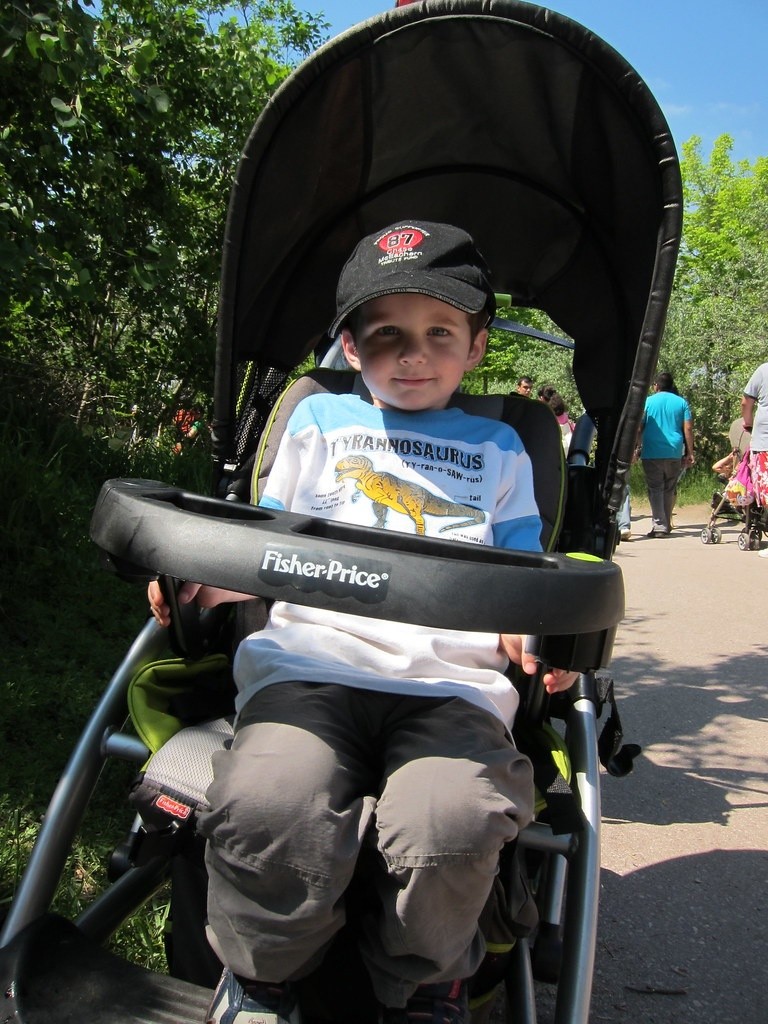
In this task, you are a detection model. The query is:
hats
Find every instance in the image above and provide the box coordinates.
[328,220,497,339]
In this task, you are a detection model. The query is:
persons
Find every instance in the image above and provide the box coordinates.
[145,217,583,1024]
[517,376,575,461]
[616,373,695,540]
[712,362,768,510]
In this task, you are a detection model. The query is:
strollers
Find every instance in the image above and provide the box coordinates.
[700,426,764,552]
[1,0,683,1024]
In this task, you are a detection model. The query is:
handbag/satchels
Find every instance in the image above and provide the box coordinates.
[725,446,754,506]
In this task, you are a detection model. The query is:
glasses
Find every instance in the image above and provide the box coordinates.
[523,386,532,390]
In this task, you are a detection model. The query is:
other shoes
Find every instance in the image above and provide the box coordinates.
[759,548,768,557]
[620,529,631,541]
[666,531,670,537]
[647,530,666,537]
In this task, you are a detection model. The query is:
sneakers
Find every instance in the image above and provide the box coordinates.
[203,965,300,1024]
[377,1004,408,1024]
